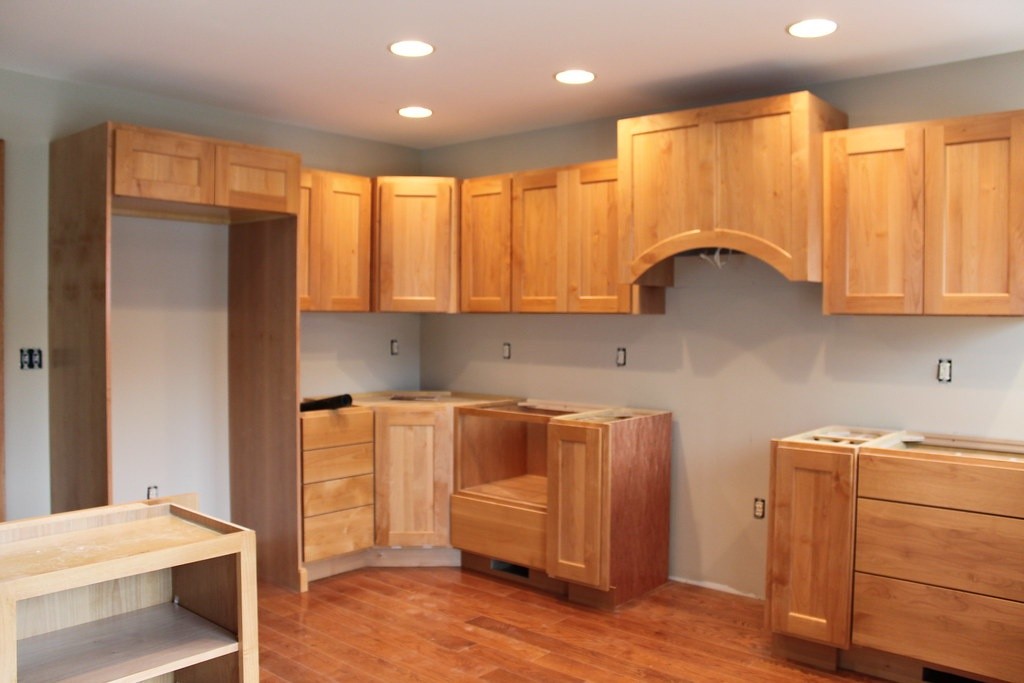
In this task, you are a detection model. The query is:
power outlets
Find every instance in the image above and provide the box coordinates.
[502,342,511,360]
[754,498,765,519]
[391,339,399,356]
[937,358,952,382]
[148,486,158,500]
[615,348,626,367]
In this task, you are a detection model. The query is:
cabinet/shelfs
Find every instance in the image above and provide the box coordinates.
[769,424,1024,683]
[46,90,1024,328]
[299,387,675,613]
[0,490,260,683]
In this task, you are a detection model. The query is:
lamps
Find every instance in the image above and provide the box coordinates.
[394,104,433,120]
[554,68,597,85]
[785,16,838,39]
[386,38,434,59]
